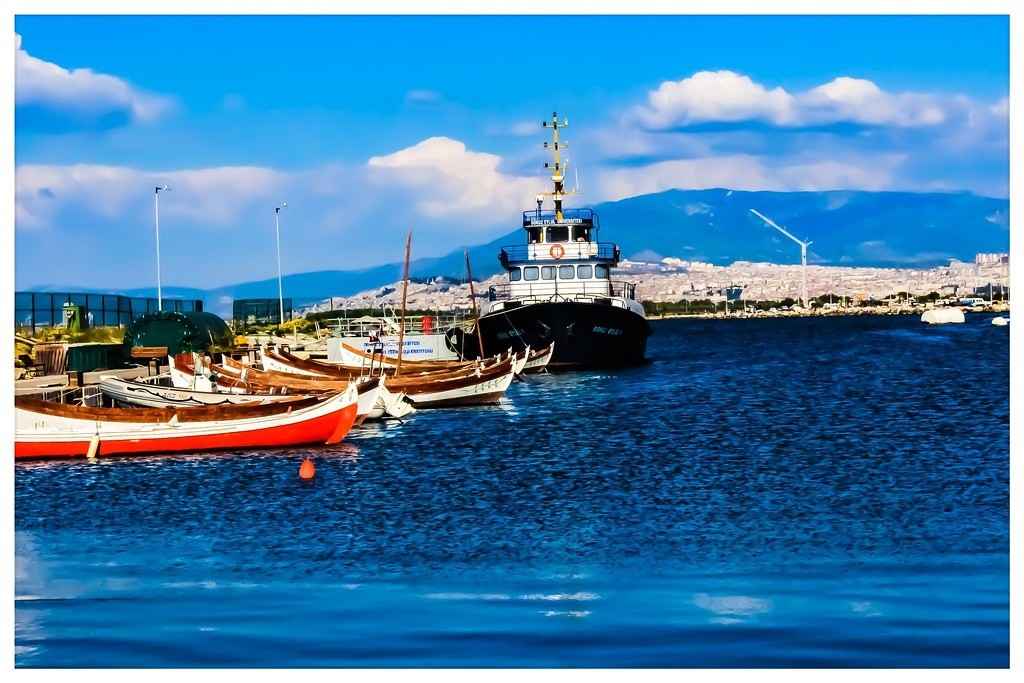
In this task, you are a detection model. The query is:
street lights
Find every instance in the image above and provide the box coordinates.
[155,185,172,312]
[276,202,286,324]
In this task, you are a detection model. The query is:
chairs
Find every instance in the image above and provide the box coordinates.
[19,355,46,378]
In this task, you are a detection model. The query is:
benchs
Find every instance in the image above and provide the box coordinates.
[130,347,168,366]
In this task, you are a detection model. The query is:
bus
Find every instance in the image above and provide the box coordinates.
[936,300,949,304]
[959,298,985,305]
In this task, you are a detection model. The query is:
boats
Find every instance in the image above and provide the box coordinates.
[15,382,358,459]
[100,339,554,426]
[457,113,656,370]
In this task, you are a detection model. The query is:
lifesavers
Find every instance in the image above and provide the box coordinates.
[549,243,566,259]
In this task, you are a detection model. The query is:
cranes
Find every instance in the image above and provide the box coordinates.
[749,211,812,308]
